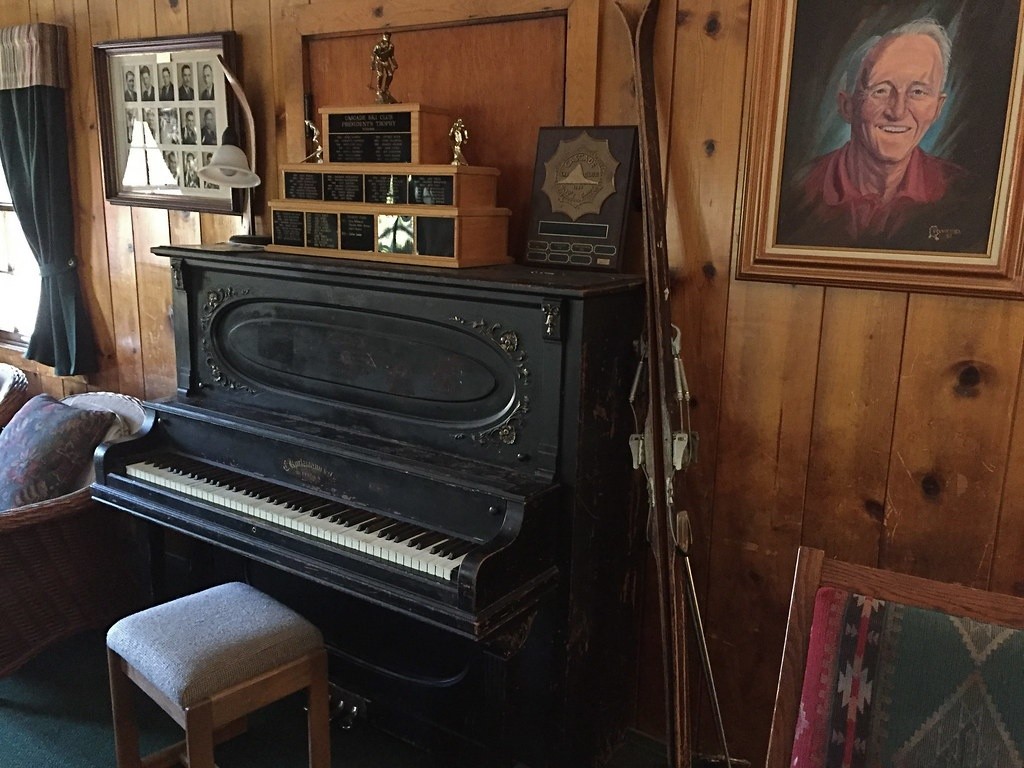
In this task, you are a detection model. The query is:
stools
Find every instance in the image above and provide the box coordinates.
[106,579,333,768]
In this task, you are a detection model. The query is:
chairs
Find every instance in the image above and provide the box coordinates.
[1,361,29,434]
[764,545,1024,768]
[0,391,147,683]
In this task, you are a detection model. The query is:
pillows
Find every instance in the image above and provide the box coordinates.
[1,392,117,513]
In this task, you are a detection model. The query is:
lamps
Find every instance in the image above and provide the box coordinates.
[197,124,272,245]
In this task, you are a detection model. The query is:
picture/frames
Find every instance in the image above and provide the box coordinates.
[92,30,247,216]
[736,1,1023,302]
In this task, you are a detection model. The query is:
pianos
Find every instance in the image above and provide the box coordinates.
[89,236,686,768]
